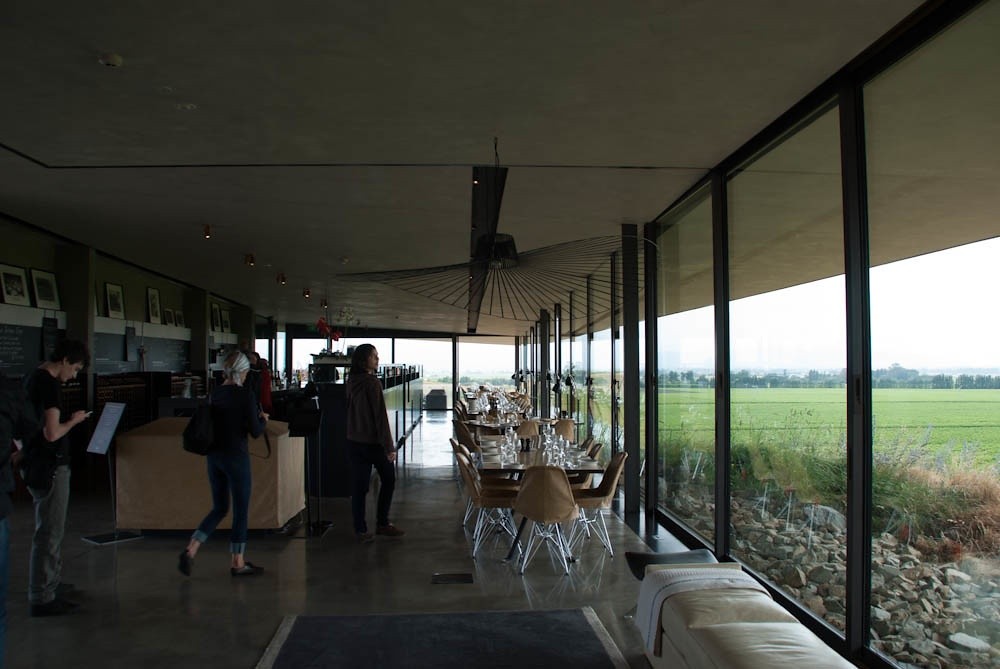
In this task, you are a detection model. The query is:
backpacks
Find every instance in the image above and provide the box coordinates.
[6,400,39,469]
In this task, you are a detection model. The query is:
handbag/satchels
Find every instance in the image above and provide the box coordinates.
[183,404,215,456]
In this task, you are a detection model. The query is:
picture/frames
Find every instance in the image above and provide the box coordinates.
[28,267,62,311]
[164,308,176,326]
[175,311,185,327]
[211,303,221,332]
[221,310,232,333]
[147,287,161,324]
[104,281,126,319]
[0,263,33,307]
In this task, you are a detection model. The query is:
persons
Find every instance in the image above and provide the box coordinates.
[179,350,272,577]
[10,340,91,617]
[348,344,407,544]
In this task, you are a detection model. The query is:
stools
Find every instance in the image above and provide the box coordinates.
[625,548,720,618]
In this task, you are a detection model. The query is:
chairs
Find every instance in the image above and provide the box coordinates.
[450,381,628,576]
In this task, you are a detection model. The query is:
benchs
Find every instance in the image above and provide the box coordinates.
[641,562,859,669]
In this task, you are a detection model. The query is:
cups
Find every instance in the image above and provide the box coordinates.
[501,439,506,452]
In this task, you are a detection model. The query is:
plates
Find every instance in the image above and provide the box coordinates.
[577,458,600,464]
[570,443,578,448]
[504,463,525,467]
[569,451,587,454]
[481,446,498,449]
[478,439,497,443]
[481,461,499,465]
[481,454,499,457]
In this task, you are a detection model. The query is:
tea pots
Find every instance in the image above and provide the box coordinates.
[319,348,331,356]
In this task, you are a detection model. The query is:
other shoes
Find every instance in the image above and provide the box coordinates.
[178,550,194,576]
[31,597,80,617]
[352,527,376,543]
[230,561,265,578]
[375,524,405,537]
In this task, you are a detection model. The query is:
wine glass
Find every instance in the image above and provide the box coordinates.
[542,422,570,466]
[505,426,522,465]
[476,388,521,426]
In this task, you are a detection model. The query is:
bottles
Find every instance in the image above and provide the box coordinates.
[271,370,302,389]
[519,437,531,452]
[382,364,408,377]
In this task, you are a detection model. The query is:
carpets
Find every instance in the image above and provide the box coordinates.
[254,604,632,669]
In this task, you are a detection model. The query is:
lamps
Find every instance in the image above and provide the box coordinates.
[281,277,287,285]
[247,255,254,266]
[305,288,311,298]
[204,223,210,239]
[323,300,330,309]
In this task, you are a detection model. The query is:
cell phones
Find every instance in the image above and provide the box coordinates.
[85,409,94,414]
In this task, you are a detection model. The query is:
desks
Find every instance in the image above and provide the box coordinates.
[461,415,558,434]
[479,432,604,560]
